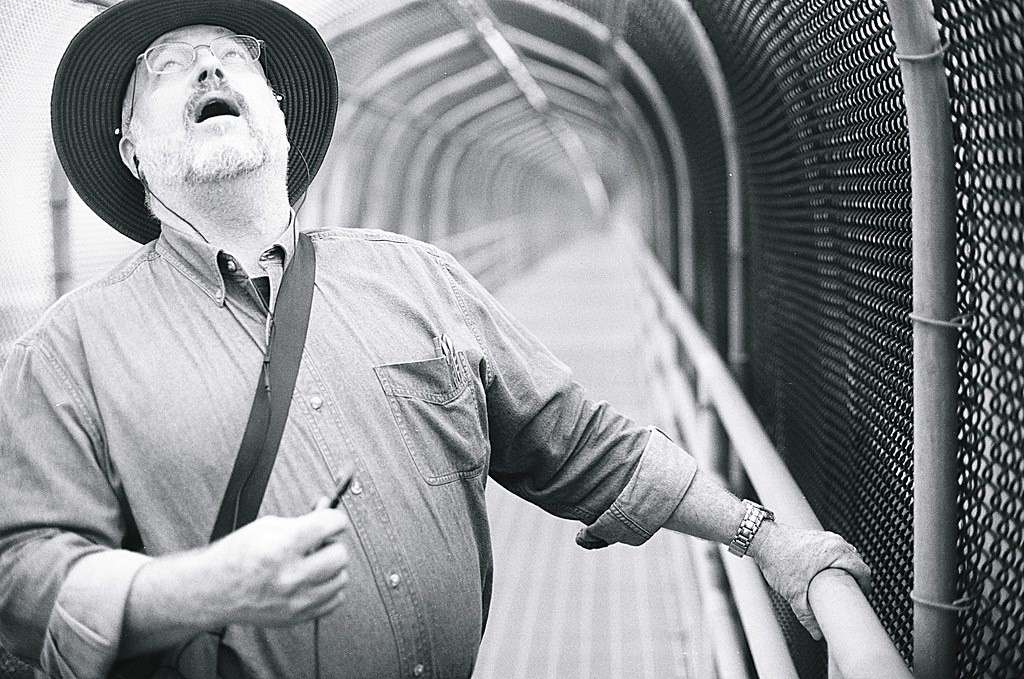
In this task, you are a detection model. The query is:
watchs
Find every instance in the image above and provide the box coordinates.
[728,499,776,559]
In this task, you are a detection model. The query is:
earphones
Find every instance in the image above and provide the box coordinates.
[133,154,140,167]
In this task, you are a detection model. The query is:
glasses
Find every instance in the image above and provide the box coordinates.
[130,35,270,126]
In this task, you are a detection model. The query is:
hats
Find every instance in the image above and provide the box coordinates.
[51,0,339,245]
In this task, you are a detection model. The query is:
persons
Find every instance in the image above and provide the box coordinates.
[1,1,874,678]
